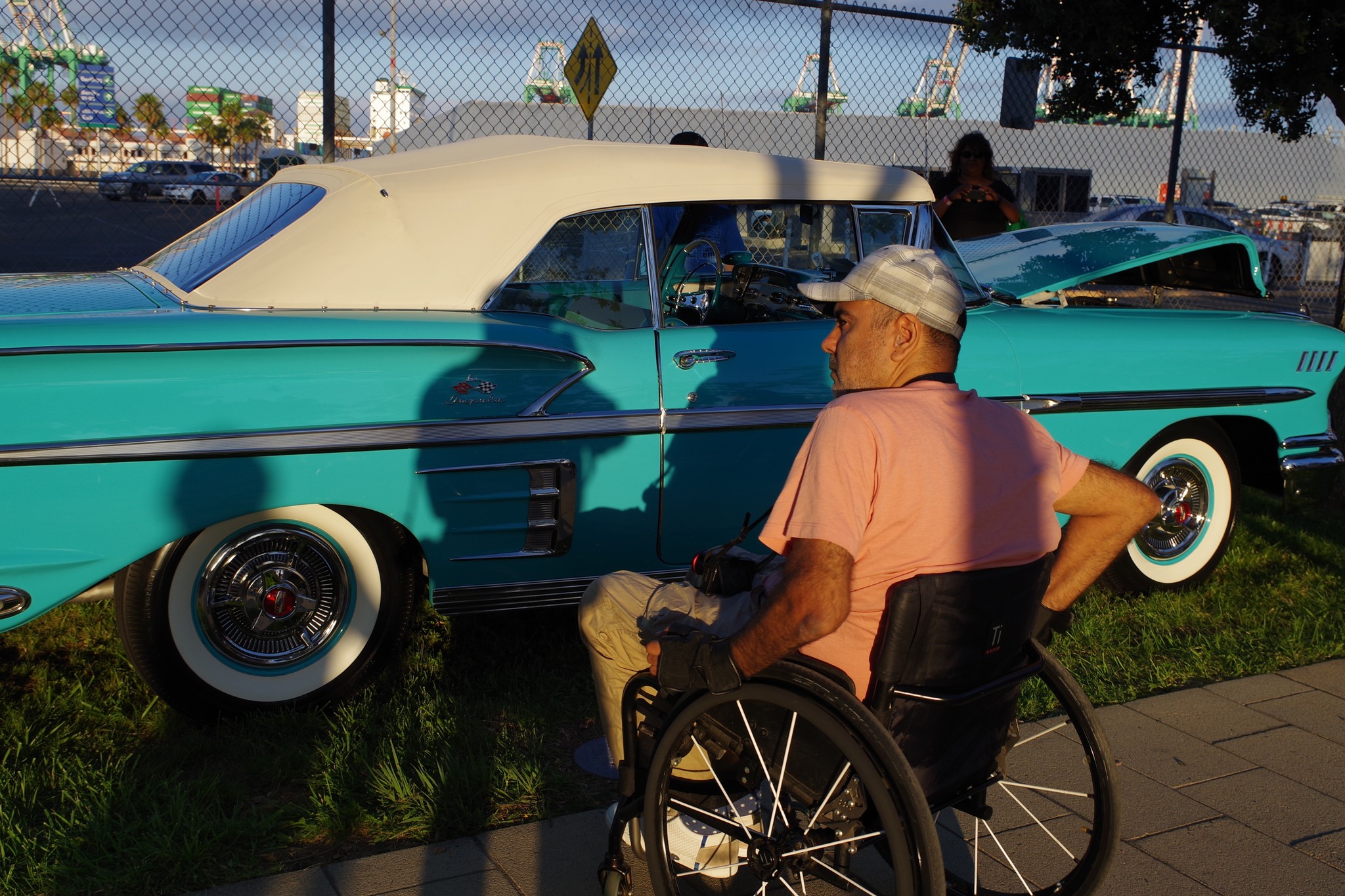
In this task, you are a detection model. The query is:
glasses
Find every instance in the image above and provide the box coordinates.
[959,151,986,159]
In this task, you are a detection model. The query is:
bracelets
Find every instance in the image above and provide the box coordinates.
[943,195,953,206]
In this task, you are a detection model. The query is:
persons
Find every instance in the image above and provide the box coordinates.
[574,243,1165,882]
[930,131,1021,249]
[650,131,749,277]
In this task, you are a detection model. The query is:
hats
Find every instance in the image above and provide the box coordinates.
[796,244,967,342]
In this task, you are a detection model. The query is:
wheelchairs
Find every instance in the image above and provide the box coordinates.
[596,549,1122,895]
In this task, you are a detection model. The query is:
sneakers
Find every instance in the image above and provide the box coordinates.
[605,797,739,878]
[731,809,765,858]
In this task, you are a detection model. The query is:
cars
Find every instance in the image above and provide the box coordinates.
[2,134,1345,730]
[1073,195,1344,288]
[93,147,315,206]
